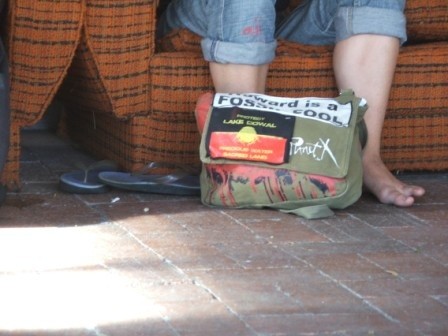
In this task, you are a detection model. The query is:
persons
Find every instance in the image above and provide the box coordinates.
[157,0,425,206]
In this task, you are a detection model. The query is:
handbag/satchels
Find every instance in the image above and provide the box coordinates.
[200,89,367,219]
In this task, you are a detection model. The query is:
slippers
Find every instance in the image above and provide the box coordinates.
[59,159,201,195]
